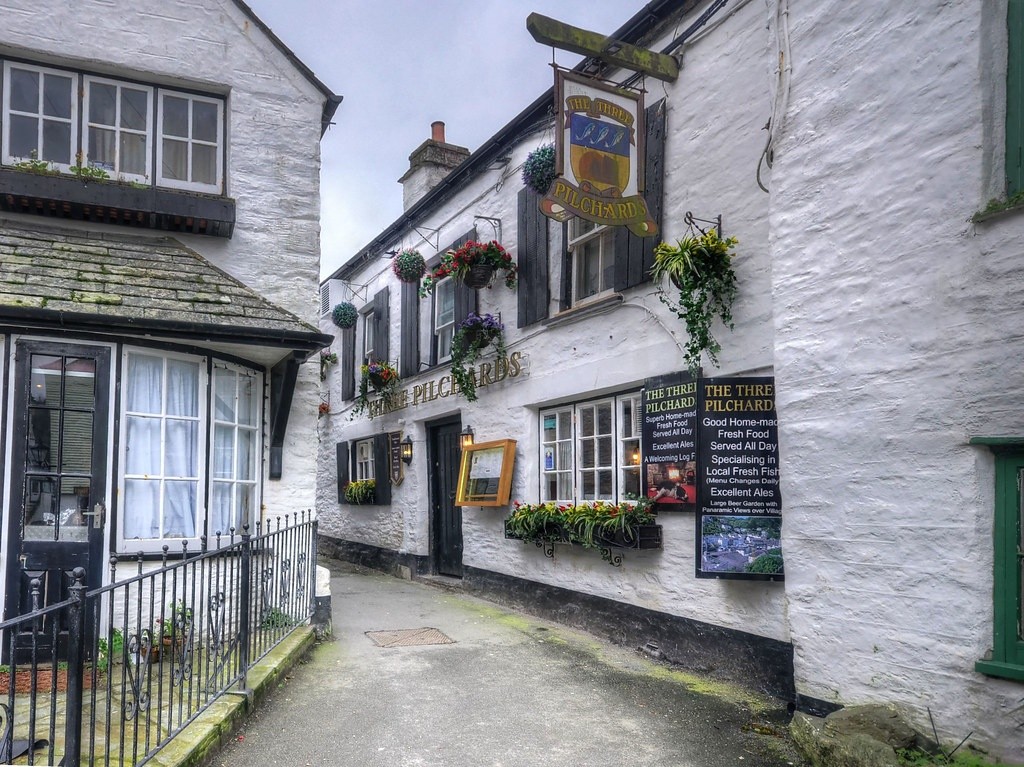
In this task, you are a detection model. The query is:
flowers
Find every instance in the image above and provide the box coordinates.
[505,491,656,562]
[649,227,745,379]
[350,355,400,421]
[342,479,375,506]
[448,313,507,403]
[418,241,519,299]
[156,598,192,639]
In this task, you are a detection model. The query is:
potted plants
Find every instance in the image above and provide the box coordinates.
[141,628,160,664]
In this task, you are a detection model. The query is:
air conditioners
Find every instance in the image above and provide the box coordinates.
[320,279,351,320]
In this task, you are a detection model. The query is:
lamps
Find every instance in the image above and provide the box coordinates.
[458,424,474,453]
[400,436,413,466]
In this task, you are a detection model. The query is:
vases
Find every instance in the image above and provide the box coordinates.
[671,263,710,289]
[162,635,188,656]
[466,333,495,348]
[371,376,389,386]
[457,264,492,290]
